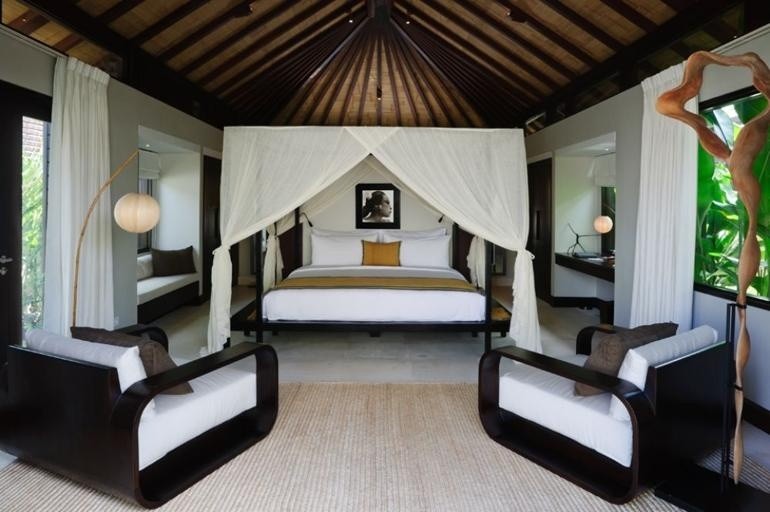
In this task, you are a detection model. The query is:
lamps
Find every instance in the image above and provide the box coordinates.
[438,214,445,223]
[300,212,312,227]
[72,150,161,326]
[566,215,614,255]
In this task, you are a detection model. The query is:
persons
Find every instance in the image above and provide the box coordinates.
[361,191,392,223]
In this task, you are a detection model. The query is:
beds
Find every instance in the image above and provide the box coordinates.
[231,265,512,338]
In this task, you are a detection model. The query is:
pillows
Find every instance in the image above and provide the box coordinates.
[23,327,155,414]
[572,321,679,398]
[310,228,451,269]
[150,245,197,277]
[608,324,719,420]
[69,325,194,395]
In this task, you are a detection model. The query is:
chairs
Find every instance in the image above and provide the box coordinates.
[0,323,279,510]
[477,323,735,505]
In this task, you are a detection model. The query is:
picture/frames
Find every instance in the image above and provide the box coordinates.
[356,183,401,230]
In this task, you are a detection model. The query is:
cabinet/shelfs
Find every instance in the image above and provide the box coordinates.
[525,157,553,302]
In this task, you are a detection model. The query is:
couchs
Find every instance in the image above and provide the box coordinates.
[136,252,201,325]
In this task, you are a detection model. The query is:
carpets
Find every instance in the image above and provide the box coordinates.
[0,380,770,512]
